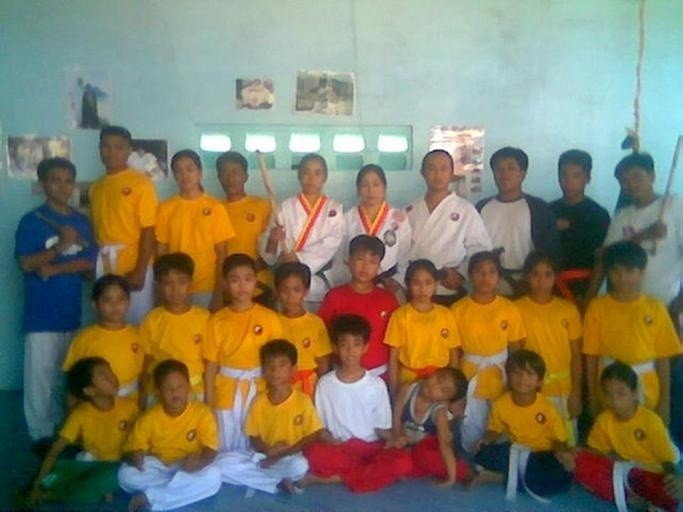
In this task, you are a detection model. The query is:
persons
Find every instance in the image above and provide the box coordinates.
[214,151,276,309]
[581,240,683,428]
[474,146,564,302]
[59,274,154,414]
[402,150,494,308]
[256,153,345,314]
[571,362,683,510]
[382,258,461,399]
[449,251,527,452]
[199,253,282,454]
[138,251,211,412]
[15,157,98,461]
[26,356,141,512]
[513,249,585,448]
[88,125,160,328]
[118,359,221,512]
[302,315,412,492]
[546,149,611,309]
[272,261,334,406]
[600,153,683,318]
[335,164,411,307]
[392,367,468,489]
[155,149,235,311]
[465,350,576,497]
[214,338,330,495]
[316,234,399,393]
